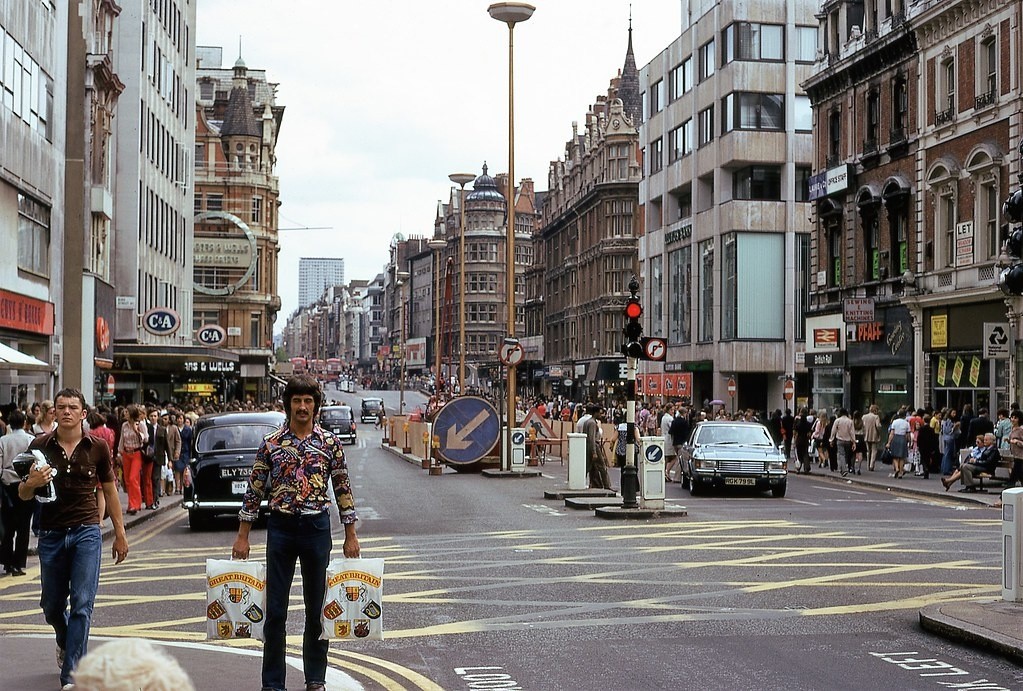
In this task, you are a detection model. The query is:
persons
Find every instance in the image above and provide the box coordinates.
[576,403,619,493]
[321,399,346,407]
[885,404,1023,506]
[375,401,385,430]
[465,385,480,396]
[361,372,436,390]
[18,387,129,691]
[197,398,286,417]
[766,405,882,476]
[232,374,360,691]
[714,404,759,423]
[605,399,708,482]
[83,401,199,529]
[0,401,58,577]
[515,393,587,422]
[440,375,450,392]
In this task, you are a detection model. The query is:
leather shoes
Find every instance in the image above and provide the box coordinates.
[306,684,325,691]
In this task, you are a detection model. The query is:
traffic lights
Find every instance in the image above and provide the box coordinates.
[212,379,234,395]
[624,296,643,358]
[1003,187,1023,297]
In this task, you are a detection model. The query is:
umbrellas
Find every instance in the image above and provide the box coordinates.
[709,400,725,405]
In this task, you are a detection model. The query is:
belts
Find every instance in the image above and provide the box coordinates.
[124,447,140,453]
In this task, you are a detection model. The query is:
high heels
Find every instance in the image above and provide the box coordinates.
[940,477,950,491]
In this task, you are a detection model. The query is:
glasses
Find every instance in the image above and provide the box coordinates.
[975,440,982,444]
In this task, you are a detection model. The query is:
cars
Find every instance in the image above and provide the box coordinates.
[182,411,287,530]
[335,374,353,389]
[319,406,357,445]
[422,395,447,422]
[679,419,788,497]
[362,398,386,423]
[370,379,388,390]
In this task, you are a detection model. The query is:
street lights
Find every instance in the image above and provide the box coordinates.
[448,173,477,396]
[305,305,329,362]
[487,1,535,470]
[398,272,410,416]
[428,240,447,410]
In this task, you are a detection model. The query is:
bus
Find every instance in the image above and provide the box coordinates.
[291,358,306,378]
[307,359,325,381]
[322,359,343,383]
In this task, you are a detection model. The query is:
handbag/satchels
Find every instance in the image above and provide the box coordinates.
[165,468,173,496]
[206,553,267,644]
[808,439,819,457]
[182,466,192,488]
[881,447,893,465]
[906,448,914,463]
[913,447,920,465]
[140,441,154,464]
[318,553,384,641]
[795,448,801,469]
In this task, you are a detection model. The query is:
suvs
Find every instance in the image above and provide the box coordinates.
[428,375,445,392]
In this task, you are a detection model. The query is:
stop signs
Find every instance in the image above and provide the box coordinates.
[107,375,115,394]
[727,379,736,396]
[784,380,794,400]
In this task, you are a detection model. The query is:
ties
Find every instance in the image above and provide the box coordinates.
[982,448,987,454]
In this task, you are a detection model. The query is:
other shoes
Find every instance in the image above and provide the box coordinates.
[3,564,12,574]
[145,504,155,510]
[12,567,26,576]
[961,488,976,493]
[665,474,673,482]
[55,642,66,669]
[943,471,952,475]
[61,683,74,690]
[674,480,682,483]
[126,510,137,516]
[795,458,930,480]
[604,486,618,492]
